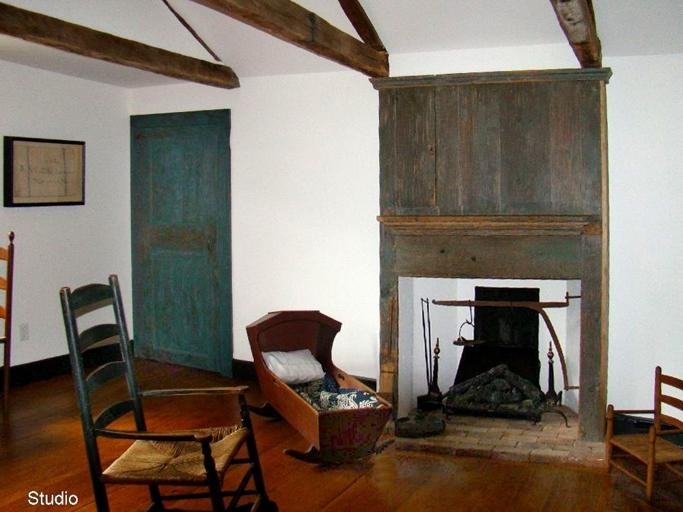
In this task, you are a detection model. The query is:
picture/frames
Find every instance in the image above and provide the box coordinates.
[3,136,86,206]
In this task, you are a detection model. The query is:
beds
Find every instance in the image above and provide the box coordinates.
[246,310,397,466]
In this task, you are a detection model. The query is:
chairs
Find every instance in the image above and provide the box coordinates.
[606,366,682,500]
[60,272,278,512]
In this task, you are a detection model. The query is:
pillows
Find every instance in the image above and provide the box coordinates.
[263,349,327,383]
